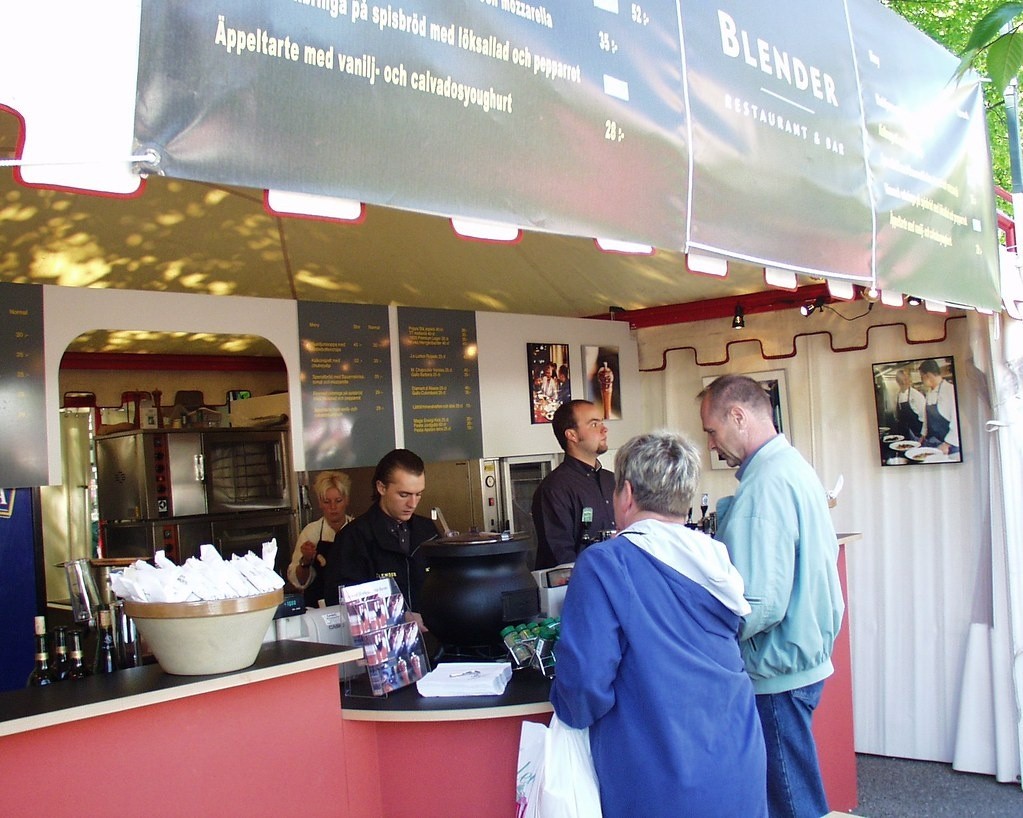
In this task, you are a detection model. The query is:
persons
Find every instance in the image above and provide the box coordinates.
[893,360,959,455]
[702,373,845,818]
[531,400,617,571]
[306,451,442,633]
[550,433,769,818]
[287,471,355,608]
[533,363,570,402]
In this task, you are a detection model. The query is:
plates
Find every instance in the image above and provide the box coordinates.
[889,440,921,452]
[904,446,943,461]
[883,434,904,443]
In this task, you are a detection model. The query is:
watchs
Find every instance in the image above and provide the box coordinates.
[299,557,311,568]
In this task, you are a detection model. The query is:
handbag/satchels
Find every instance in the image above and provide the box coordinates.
[515,712,603,818]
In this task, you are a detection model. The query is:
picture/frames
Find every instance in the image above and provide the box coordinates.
[872,356,963,467]
[527,343,572,424]
[701,368,791,470]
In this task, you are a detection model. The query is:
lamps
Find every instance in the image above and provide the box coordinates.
[732,304,745,330]
[799,296,826,317]
[907,296,922,306]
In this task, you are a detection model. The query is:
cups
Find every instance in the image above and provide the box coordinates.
[64,557,105,623]
[499,616,561,669]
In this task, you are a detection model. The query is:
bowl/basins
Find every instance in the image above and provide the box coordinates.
[123,589,285,676]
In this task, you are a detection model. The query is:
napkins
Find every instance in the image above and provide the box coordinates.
[417,662,514,698]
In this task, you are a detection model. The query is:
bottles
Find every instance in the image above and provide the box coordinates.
[94,603,120,675]
[50,624,73,680]
[67,630,91,680]
[27,616,57,688]
